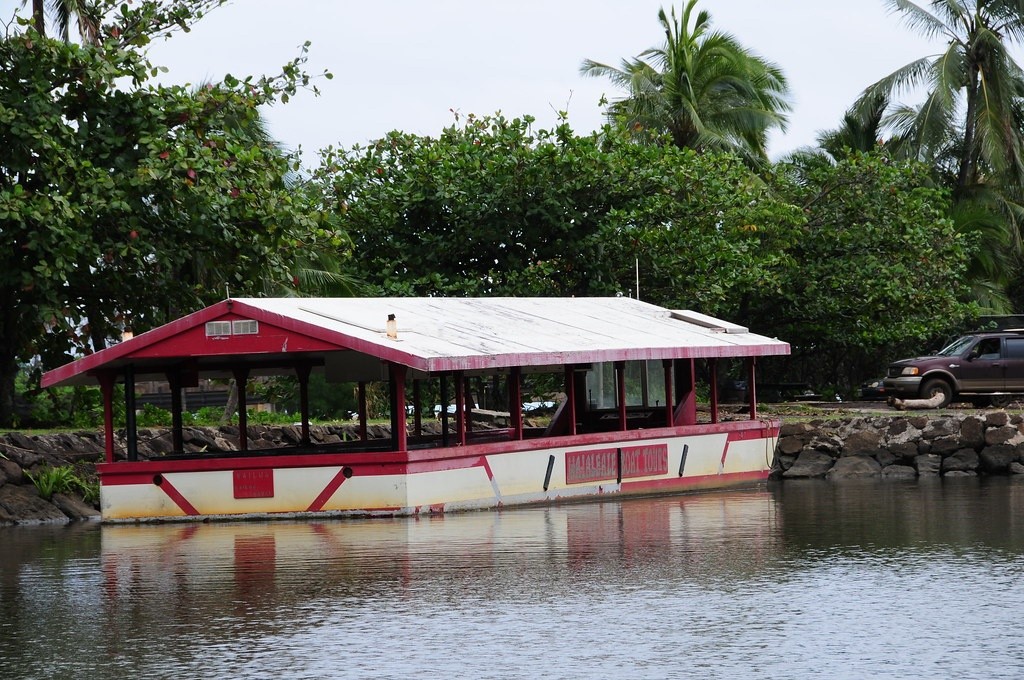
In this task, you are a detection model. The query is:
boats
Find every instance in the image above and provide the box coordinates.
[39,297,795,526]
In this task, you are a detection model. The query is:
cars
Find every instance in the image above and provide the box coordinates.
[521,401,559,419]
[432,401,479,421]
[861,373,888,401]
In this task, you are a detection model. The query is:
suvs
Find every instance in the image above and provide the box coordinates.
[882,328,1024,409]
